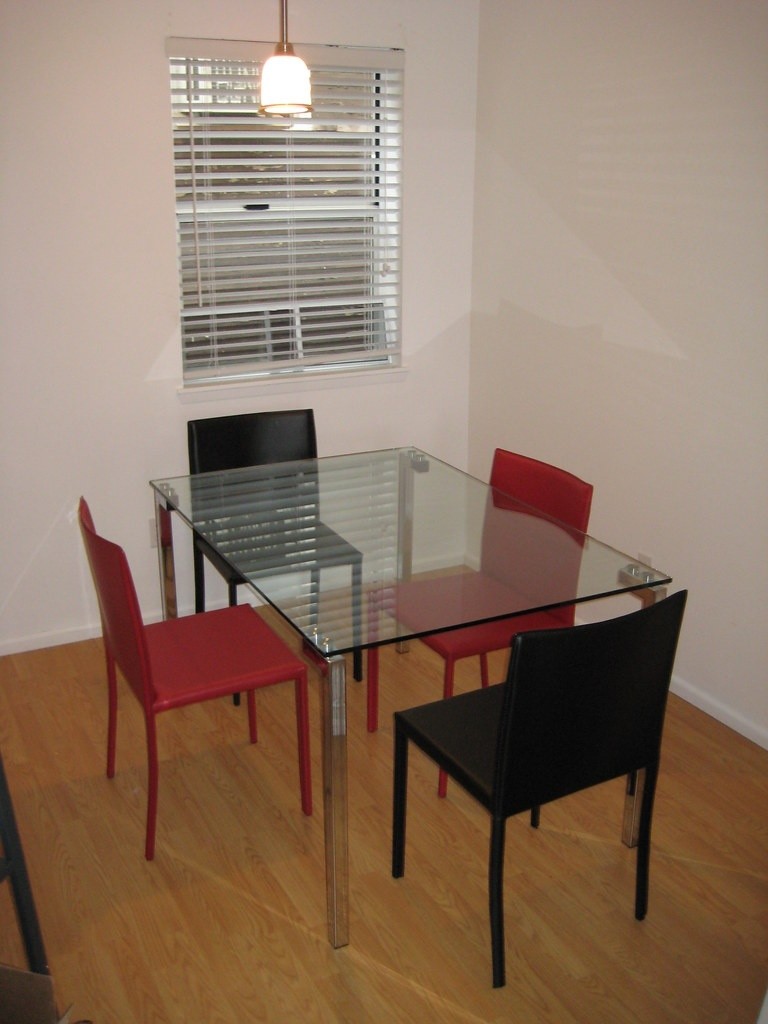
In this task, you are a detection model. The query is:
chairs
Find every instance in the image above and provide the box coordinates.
[186,408,367,706]
[76,499,312,861]
[365,451,593,796]
[389,588,692,987]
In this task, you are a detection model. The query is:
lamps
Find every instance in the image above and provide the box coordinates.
[258,1,311,113]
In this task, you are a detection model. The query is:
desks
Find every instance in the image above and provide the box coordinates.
[149,449,673,951]
